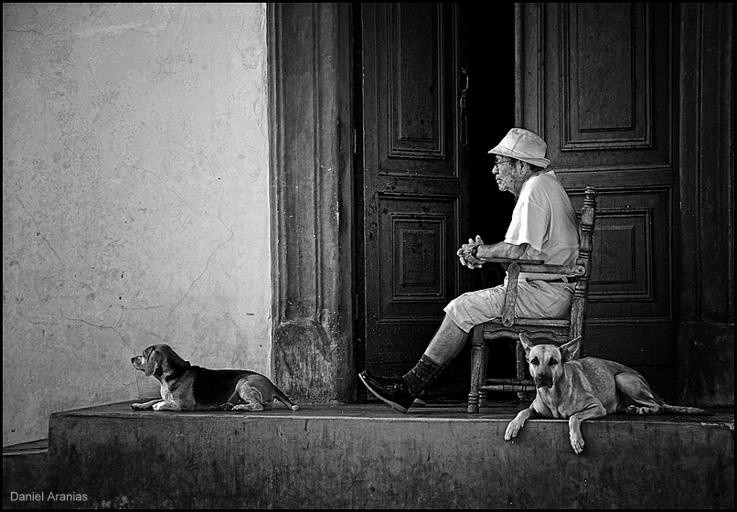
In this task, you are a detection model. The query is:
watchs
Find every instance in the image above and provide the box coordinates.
[471,244,481,260]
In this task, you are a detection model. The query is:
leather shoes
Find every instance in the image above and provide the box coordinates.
[358,371,415,414]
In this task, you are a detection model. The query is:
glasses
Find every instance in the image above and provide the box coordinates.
[495,159,512,169]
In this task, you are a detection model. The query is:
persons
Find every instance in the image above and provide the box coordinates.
[357,127,580,414]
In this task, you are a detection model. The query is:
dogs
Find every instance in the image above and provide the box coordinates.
[129,344,300,412]
[504,332,705,455]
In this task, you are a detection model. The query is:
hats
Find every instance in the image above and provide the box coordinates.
[487,127,551,169]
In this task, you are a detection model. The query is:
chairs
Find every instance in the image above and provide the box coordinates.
[465,185,600,415]
